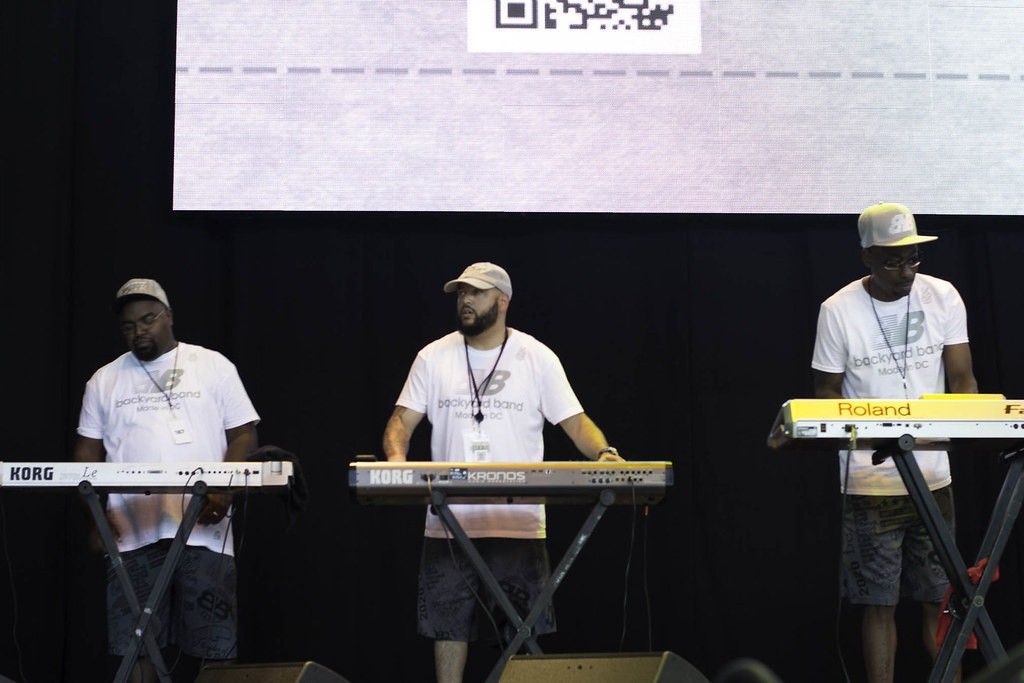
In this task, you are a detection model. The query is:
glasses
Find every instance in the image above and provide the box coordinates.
[864,249,922,271]
[119,308,168,335]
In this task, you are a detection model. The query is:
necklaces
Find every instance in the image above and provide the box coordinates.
[867,275,910,391]
[465,334,506,433]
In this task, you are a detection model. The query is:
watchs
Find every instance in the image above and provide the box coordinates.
[598,446,619,459]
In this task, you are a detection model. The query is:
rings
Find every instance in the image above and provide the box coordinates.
[213,511,221,515]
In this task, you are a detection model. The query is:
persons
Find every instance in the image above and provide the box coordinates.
[74,278,262,683]
[383,262,626,683]
[810,203,979,683]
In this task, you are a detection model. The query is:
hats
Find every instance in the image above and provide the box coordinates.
[443,262,512,301]
[116,278,170,310]
[858,201,939,249]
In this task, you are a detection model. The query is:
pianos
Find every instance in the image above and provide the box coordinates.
[0,461,294,495]
[347,456,674,507]
[767,394,1024,453]
